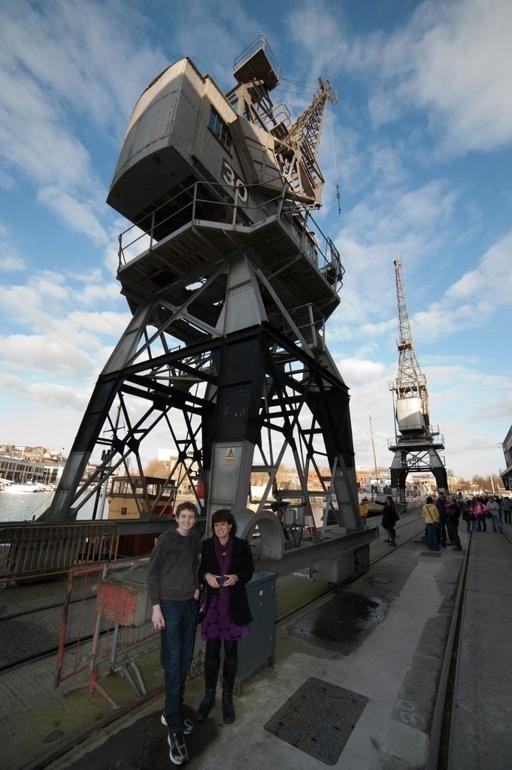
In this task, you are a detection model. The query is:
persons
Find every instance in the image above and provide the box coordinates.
[144,501,202,765]
[358,477,511,558]
[197,508,249,726]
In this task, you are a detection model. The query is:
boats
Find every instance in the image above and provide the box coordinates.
[75,389,205,563]
[0,476,104,498]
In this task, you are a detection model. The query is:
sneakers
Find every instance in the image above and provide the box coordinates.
[161,709,194,735]
[168,732,189,764]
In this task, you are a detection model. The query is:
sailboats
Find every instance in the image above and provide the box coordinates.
[321,415,433,525]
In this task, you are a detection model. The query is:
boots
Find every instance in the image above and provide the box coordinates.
[222,659,238,724]
[197,660,220,719]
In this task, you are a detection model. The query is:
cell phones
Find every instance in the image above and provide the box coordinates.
[216,576,227,586]
